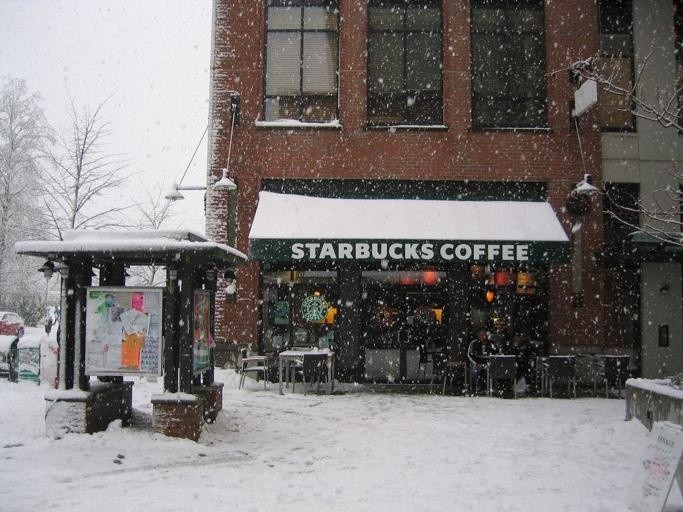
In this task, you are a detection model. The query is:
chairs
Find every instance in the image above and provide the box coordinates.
[295,353,328,395]
[414,337,631,399]
[238,348,270,392]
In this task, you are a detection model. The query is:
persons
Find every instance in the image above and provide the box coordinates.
[52,306,60,325]
[467,326,499,370]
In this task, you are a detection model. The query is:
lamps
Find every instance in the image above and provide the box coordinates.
[164,182,207,202]
[574,173,600,197]
[37,253,58,283]
[164,256,181,282]
[212,93,240,191]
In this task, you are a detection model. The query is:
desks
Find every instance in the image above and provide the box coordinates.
[278,348,336,395]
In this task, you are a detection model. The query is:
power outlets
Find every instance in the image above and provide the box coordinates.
[224,263,236,285]
